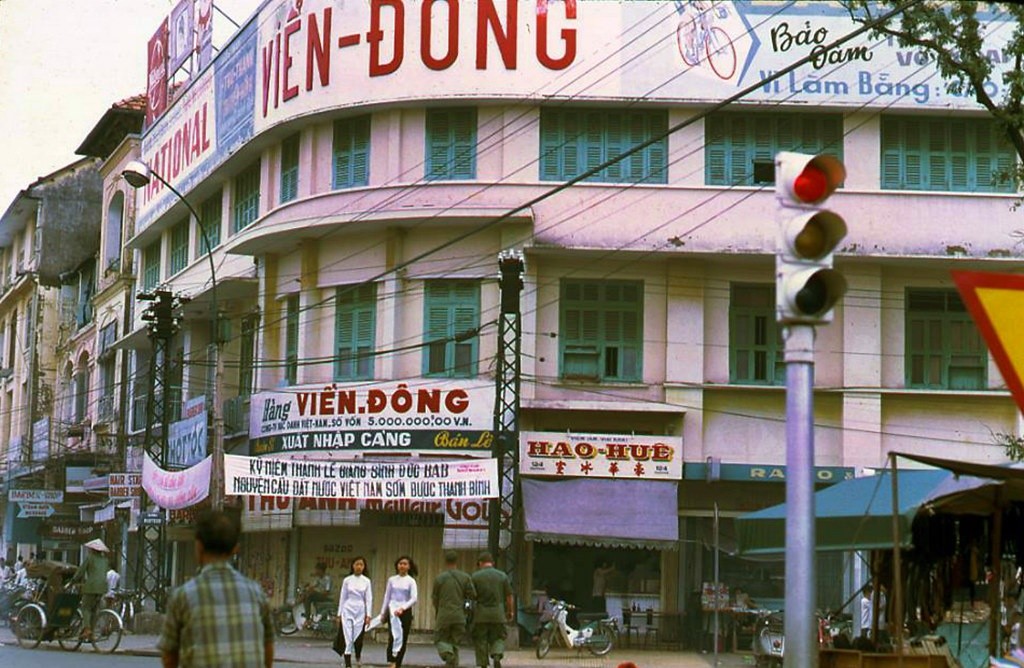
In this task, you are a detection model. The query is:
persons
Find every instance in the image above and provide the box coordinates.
[104,566,121,610]
[592,560,615,614]
[861,585,886,640]
[63,539,110,643]
[377,555,419,668]
[431,551,477,668]
[333,556,372,668]
[300,562,331,627]
[0,552,41,600]
[470,551,514,668]
[154,510,277,668]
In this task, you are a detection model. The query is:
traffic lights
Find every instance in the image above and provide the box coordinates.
[774,149,848,325]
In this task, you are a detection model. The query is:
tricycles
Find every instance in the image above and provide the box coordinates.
[13,560,125,655]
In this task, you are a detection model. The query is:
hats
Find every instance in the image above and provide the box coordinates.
[444,550,457,562]
[84,538,110,553]
[477,551,493,561]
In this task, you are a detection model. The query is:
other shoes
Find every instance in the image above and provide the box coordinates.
[494,655,501,668]
[445,655,455,668]
[80,628,91,637]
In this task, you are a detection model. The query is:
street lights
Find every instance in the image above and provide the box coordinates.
[123,159,226,507]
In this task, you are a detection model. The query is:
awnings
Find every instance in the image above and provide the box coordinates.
[518,475,680,553]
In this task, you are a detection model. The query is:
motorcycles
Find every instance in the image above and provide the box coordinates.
[534,598,620,660]
[275,586,341,641]
